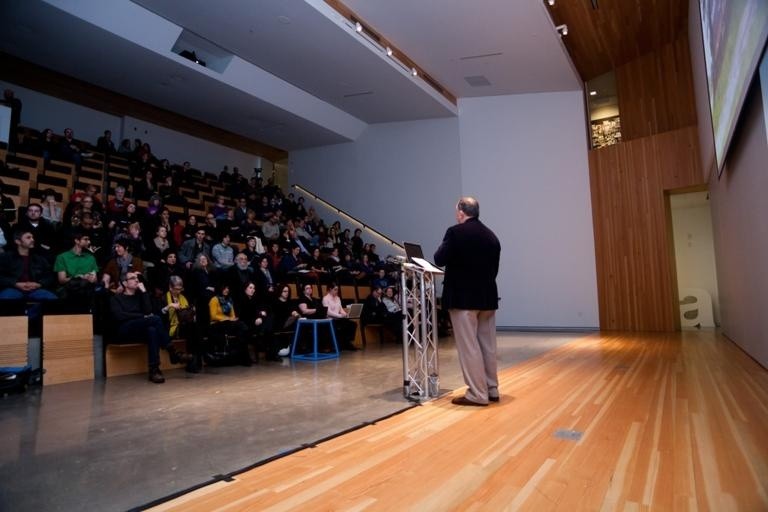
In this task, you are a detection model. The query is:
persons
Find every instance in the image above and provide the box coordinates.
[434,196,500,404]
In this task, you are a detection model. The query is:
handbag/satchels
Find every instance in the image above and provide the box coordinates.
[175,307,196,322]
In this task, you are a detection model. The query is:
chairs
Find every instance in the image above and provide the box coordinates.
[0,126,218,394]
[219,175,452,367]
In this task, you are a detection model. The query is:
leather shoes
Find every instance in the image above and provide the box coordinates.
[147,368,165,385]
[170,350,195,365]
[451,395,499,407]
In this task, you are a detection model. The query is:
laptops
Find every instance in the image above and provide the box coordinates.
[403,241,445,273]
[343,303,364,318]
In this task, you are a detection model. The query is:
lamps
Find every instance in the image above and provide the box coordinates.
[555,24,568,35]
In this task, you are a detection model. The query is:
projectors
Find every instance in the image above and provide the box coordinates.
[180,50,206,67]
